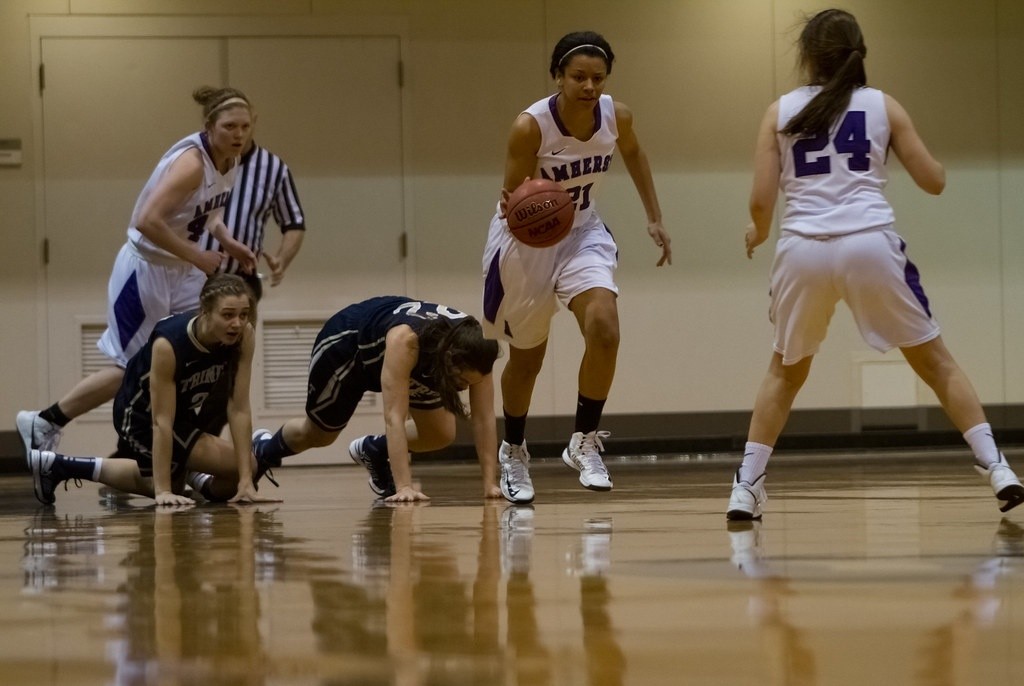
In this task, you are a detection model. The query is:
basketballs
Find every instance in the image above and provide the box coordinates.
[506,179,575,248]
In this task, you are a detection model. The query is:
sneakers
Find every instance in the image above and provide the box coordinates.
[252,428,278,487]
[562,430,614,490]
[727,470,767,519]
[348,435,395,496]
[973,451,1024,512]
[499,439,534,505]
[31,449,62,504]
[16,409,62,470]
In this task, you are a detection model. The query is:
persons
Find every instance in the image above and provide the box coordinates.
[724,8,1024,520]
[21,508,1024,686]
[251,295,500,502]
[482,31,672,504]
[15,85,305,507]
